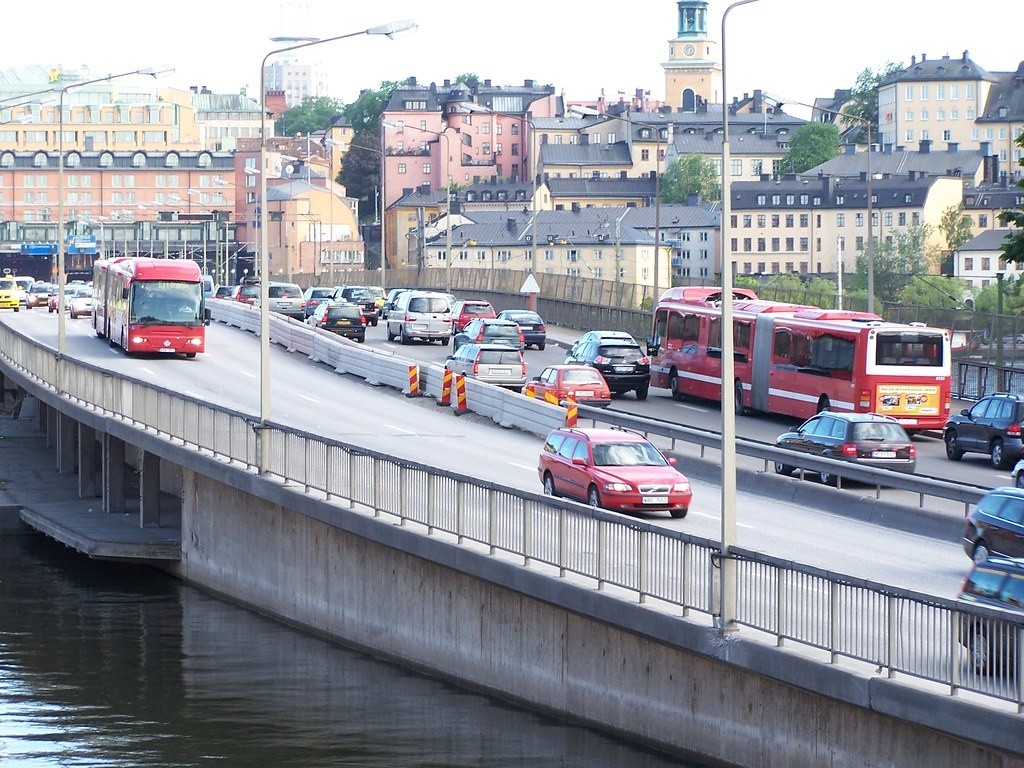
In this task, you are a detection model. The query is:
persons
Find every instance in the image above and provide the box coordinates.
[178,300,193,313]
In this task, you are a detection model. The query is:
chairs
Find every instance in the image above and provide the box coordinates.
[880,357,897,363]
[898,358,913,363]
[915,358,930,364]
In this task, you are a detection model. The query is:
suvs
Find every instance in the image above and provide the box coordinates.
[962,486,1024,567]
[942,392,1024,471]
[774,410,918,489]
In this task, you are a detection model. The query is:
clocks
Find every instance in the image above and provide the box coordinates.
[682,44,696,56]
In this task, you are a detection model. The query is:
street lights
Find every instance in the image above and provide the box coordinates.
[111,212,128,257]
[139,205,169,258]
[152,200,187,259]
[171,196,206,274]
[258,17,419,471]
[88,218,105,258]
[483,106,537,314]
[188,189,229,286]
[324,138,385,288]
[215,179,258,277]
[570,104,660,309]
[761,91,874,314]
[380,118,451,291]
[281,147,334,287]
[123,209,140,258]
[98,215,115,258]
[245,165,293,284]
[57,64,176,348]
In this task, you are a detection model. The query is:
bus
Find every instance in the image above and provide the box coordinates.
[91,255,212,358]
[645,286,952,437]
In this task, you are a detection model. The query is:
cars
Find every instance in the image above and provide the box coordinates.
[14,276,93,313]
[70,287,92,319]
[538,423,693,519]
[1003,332,1024,344]
[203,273,651,418]
[0,274,23,312]
[954,555,1024,680]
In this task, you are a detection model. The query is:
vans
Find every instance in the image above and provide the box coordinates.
[386,290,454,346]
